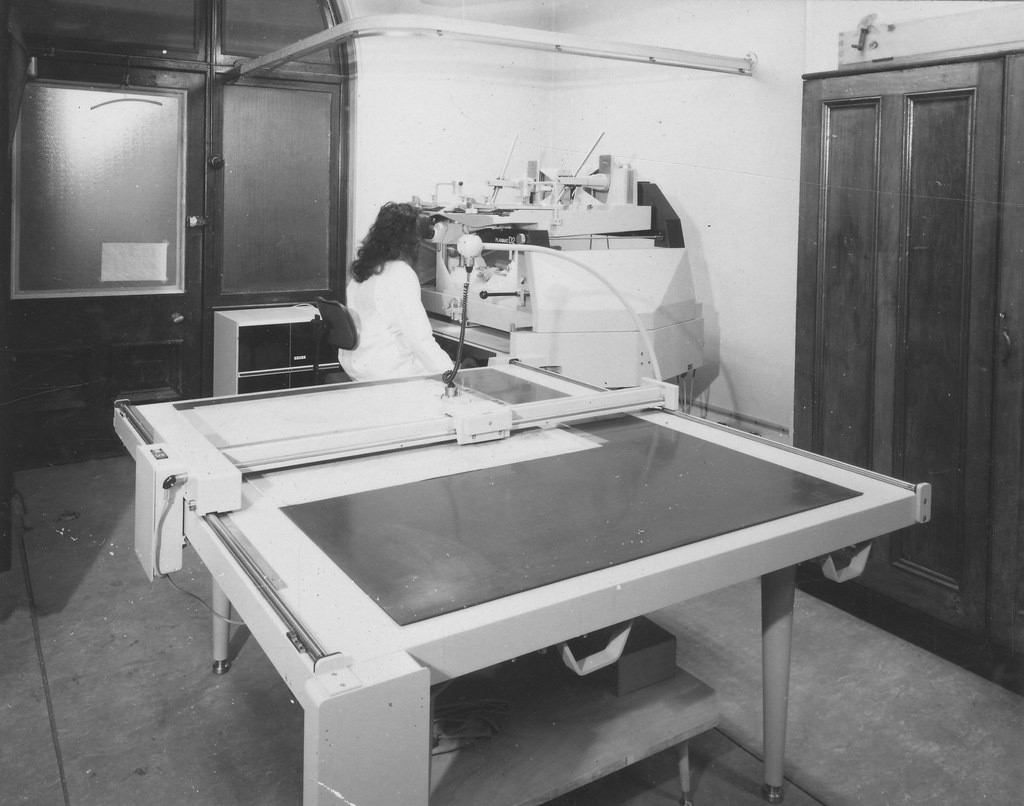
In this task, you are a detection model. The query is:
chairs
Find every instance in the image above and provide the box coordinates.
[314,296,359,389]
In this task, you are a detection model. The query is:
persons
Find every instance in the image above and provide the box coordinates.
[335,201,456,381]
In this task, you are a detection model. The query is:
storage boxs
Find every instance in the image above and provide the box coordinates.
[561,615,677,696]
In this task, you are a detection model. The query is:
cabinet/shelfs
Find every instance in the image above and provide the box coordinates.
[213,308,325,396]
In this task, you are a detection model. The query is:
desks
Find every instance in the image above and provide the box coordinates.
[109,360,934,806]
